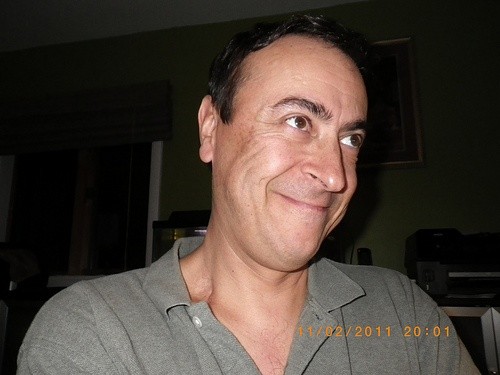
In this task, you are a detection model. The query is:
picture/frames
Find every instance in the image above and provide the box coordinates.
[357,36,427,170]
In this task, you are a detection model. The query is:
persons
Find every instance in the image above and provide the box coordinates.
[16,12,481,375]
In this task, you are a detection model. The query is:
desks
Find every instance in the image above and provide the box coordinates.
[48,276,500,375]
